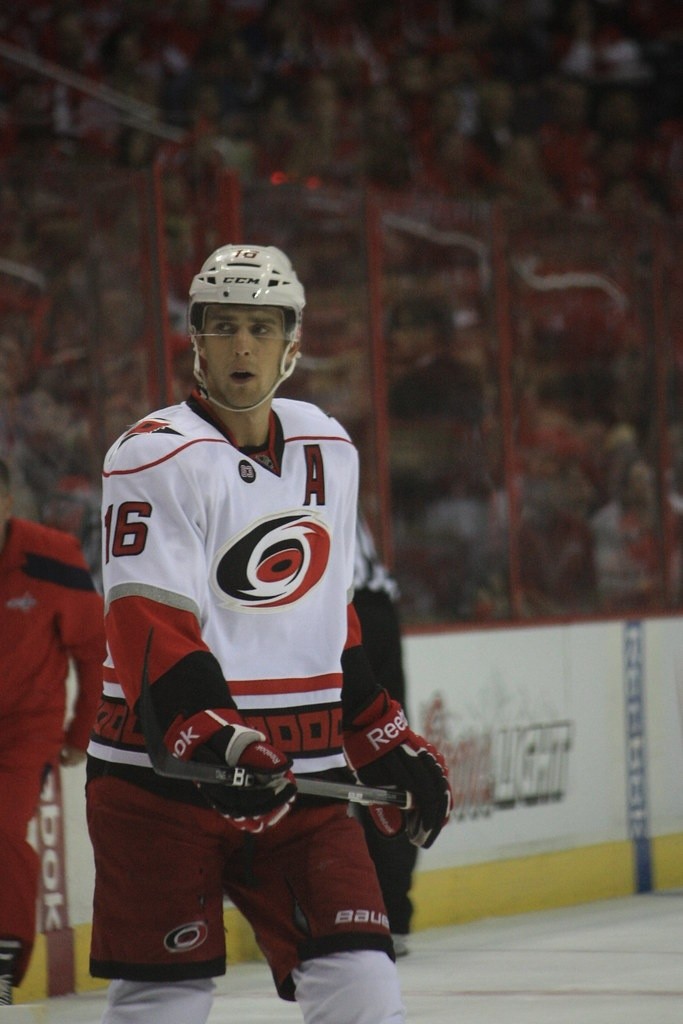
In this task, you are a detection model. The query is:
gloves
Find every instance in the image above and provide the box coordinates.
[161,704,297,835]
[340,682,455,849]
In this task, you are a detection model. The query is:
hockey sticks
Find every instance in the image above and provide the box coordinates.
[138,626,416,810]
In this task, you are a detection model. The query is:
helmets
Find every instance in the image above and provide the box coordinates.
[186,243,308,308]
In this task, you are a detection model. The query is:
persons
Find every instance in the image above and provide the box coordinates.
[357,522,421,957]
[2,455,103,1009]
[89,241,456,1024]
[1,2,680,647]
